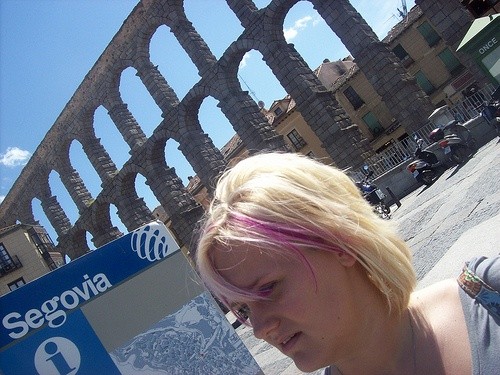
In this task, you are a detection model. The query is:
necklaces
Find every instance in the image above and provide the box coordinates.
[336,308,417,375]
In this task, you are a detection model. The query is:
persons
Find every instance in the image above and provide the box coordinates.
[194,148,500,375]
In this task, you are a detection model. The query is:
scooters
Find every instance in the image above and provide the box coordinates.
[401,135,446,186]
[428,115,479,164]
[353,165,392,219]
[473,86,500,136]
